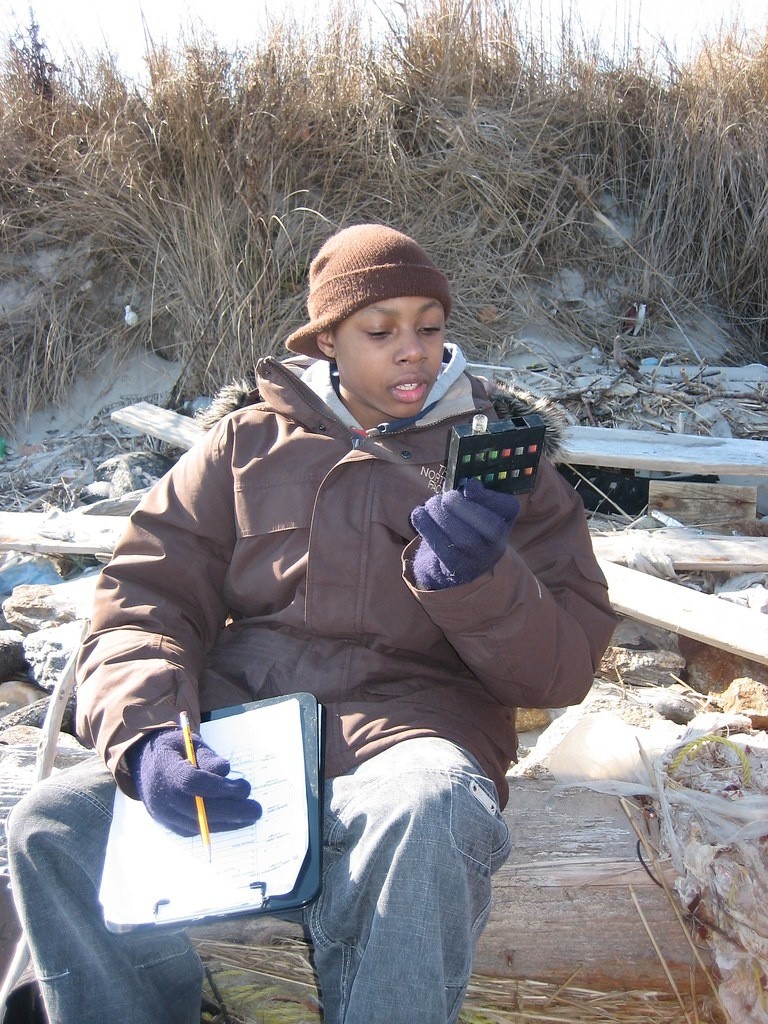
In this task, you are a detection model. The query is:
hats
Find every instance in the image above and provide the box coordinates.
[285,224,452,364]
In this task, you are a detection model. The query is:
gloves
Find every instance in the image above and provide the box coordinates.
[125,726,262,837]
[410,478,520,591]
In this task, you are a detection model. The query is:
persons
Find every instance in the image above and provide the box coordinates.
[5,224,623,1024]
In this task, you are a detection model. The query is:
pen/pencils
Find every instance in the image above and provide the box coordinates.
[179,709,213,864]
